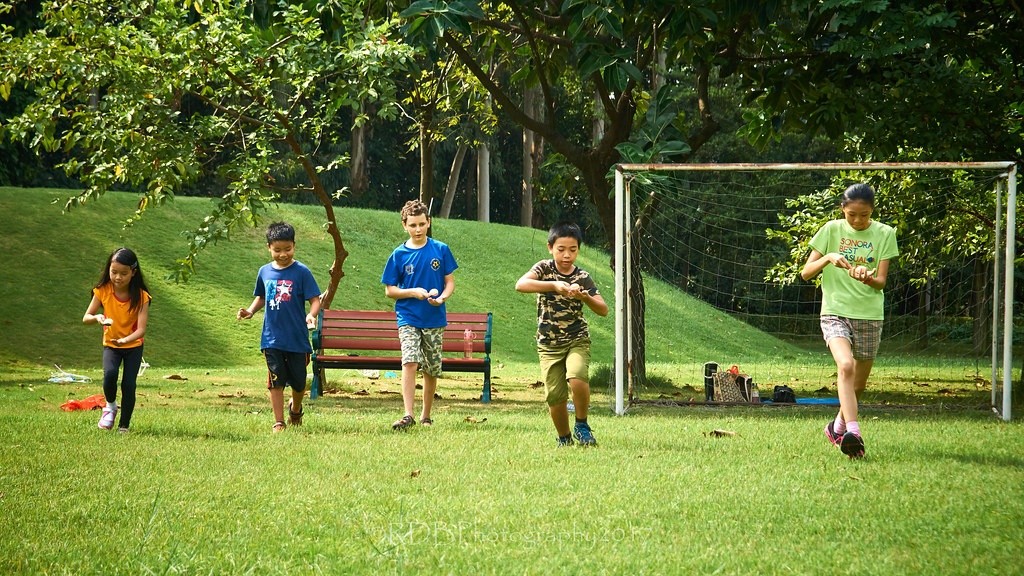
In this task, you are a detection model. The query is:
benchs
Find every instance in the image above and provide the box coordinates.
[311,310,492,404]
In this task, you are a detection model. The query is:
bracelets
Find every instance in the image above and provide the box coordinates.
[863,275,873,284]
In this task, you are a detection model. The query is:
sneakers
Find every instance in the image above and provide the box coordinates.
[288,396,304,426]
[98,407,117,429]
[560,435,573,445]
[825,421,847,448]
[840,431,864,460]
[272,421,286,432]
[573,421,595,444]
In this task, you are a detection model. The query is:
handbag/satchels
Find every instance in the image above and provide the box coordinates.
[773,385,796,402]
[713,371,746,402]
[737,373,752,402]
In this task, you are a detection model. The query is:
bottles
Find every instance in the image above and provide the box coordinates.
[463,328,477,358]
[567,404,576,412]
[751,383,759,402]
[701,361,722,400]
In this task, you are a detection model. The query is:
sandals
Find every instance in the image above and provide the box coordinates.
[393,415,416,428]
[420,418,431,427]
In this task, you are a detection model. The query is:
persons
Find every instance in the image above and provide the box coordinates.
[801,184,899,460]
[380,199,459,431]
[514,221,609,448]
[237,221,322,432]
[83,248,152,435]
